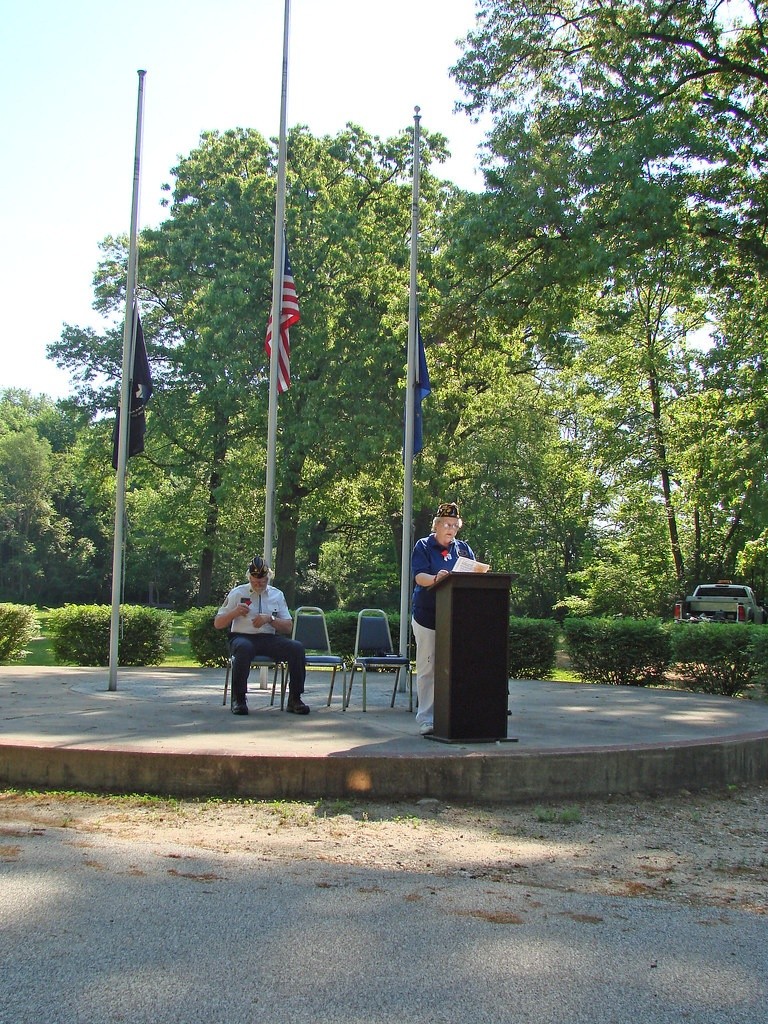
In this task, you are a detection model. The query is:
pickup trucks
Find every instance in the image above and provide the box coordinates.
[671,579,768,625]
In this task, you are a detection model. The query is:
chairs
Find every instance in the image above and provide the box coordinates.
[223,627,284,711]
[285,607,346,711]
[346,609,412,712]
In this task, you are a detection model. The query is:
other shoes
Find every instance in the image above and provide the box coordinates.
[231,697,248,715]
[286,696,310,714]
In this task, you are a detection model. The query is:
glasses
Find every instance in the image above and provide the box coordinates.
[439,520,459,529]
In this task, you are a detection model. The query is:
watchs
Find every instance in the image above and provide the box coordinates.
[269,614,275,625]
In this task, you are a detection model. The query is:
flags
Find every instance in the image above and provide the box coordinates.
[264,222,301,395]
[403,301,430,465]
[112,304,153,472]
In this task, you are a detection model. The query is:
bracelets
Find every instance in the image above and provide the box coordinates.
[434,575,438,583]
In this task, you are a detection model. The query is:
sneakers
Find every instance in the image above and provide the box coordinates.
[418,722,433,735]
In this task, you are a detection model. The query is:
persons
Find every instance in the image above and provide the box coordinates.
[213,557,310,715]
[411,502,476,733]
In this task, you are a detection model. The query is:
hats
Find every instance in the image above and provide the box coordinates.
[434,503,459,518]
[249,557,268,578]
